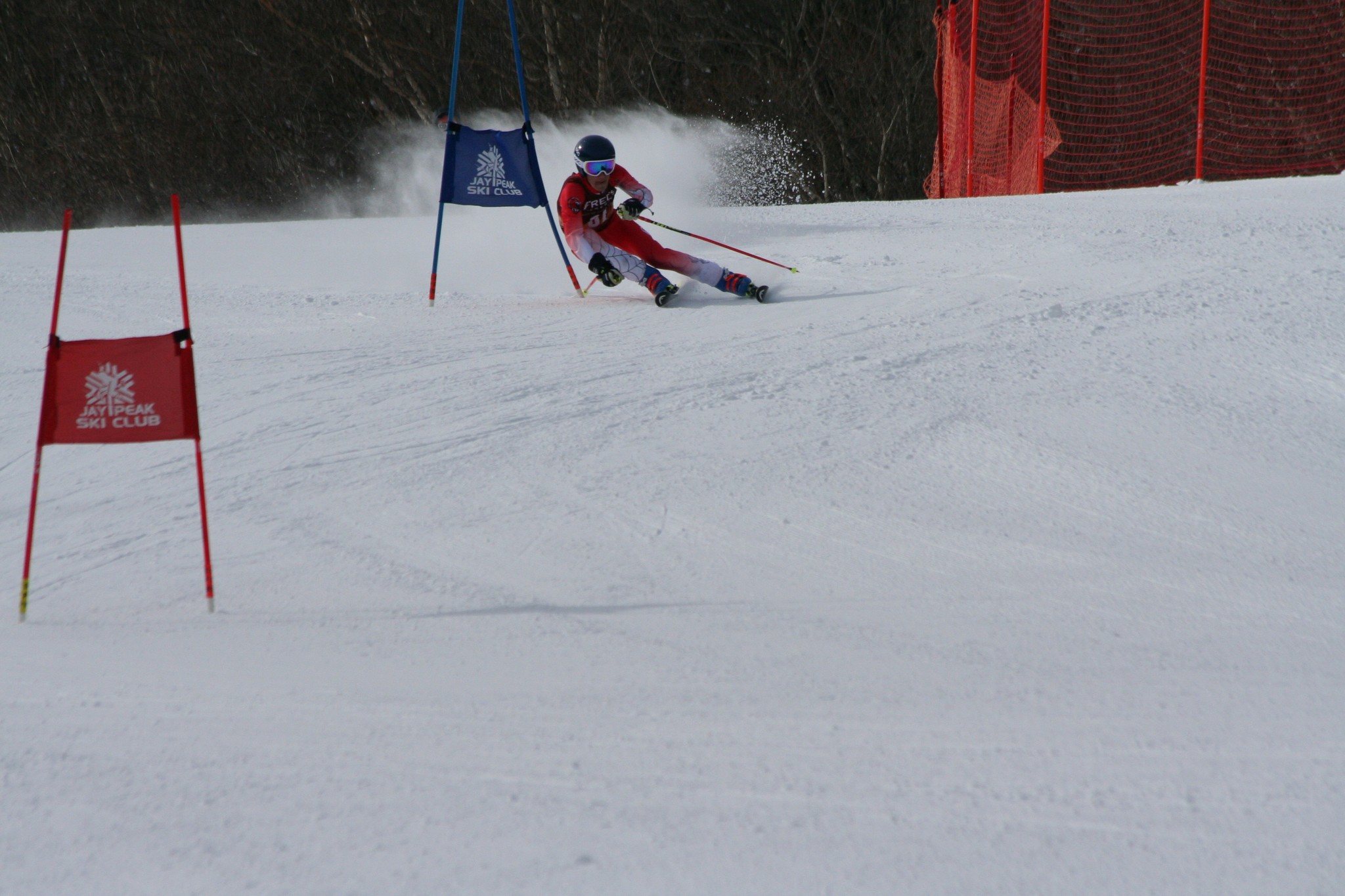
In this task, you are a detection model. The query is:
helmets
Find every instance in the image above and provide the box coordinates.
[576,135,616,178]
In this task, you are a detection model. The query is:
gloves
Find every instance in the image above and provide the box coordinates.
[587,252,624,288]
[618,198,642,221]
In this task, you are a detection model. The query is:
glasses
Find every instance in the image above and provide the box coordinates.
[573,154,615,176]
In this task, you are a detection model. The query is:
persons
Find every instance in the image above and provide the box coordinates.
[555,134,769,307]
[434,103,463,144]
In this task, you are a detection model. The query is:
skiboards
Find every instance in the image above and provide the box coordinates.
[654,285,771,307]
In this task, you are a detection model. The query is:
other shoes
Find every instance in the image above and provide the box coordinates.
[639,265,671,297]
[715,267,750,297]
